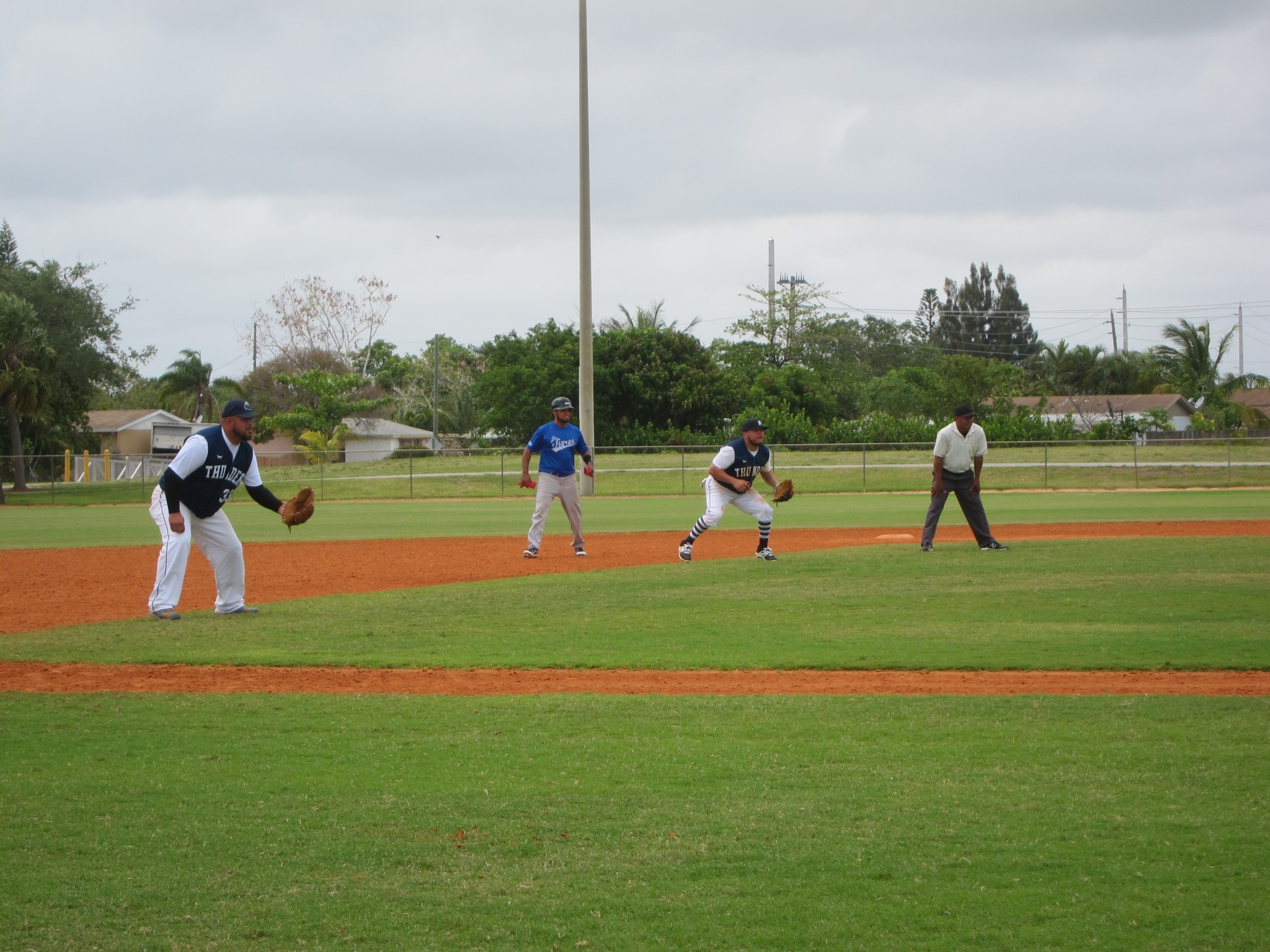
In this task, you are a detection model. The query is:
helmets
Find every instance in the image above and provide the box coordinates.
[552,397,575,411]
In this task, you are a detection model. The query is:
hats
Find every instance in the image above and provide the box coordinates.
[955,403,975,420]
[742,418,769,432]
[221,399,260,418]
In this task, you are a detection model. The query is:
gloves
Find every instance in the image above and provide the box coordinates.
[584,466,594,478]
[700,477,707,488]
[518,479,536,489]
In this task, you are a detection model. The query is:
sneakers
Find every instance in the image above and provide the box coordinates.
[920,540,932,551]
[755,544,776,560]
[678,538,692,562]
[148,606,181,621]
[575,546,586,555]
[523,547,538,558]
[981,540,1008,550]
[236,605,258,614]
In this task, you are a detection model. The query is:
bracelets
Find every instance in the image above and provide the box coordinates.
[774,483,780,490]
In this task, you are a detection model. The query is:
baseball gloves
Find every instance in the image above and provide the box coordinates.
[771,478,795,504]
[282,486,315,526]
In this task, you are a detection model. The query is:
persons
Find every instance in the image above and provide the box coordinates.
[148,398,315,621]
[920,404,1009,552]
[678,418,794,562]
[522,396,594,558]
